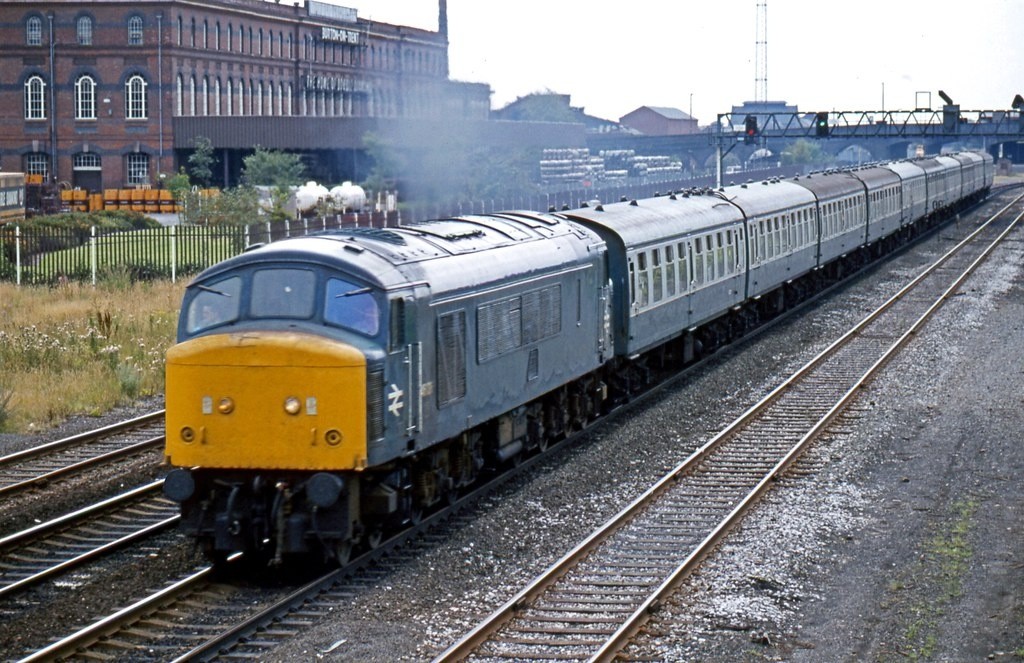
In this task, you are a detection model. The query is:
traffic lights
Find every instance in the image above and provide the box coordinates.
[745,115,759,136]
[817,112,834,139]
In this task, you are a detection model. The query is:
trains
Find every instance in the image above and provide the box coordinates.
[161,151,994,591]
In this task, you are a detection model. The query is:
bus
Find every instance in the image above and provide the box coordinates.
[0,172,27,225]
